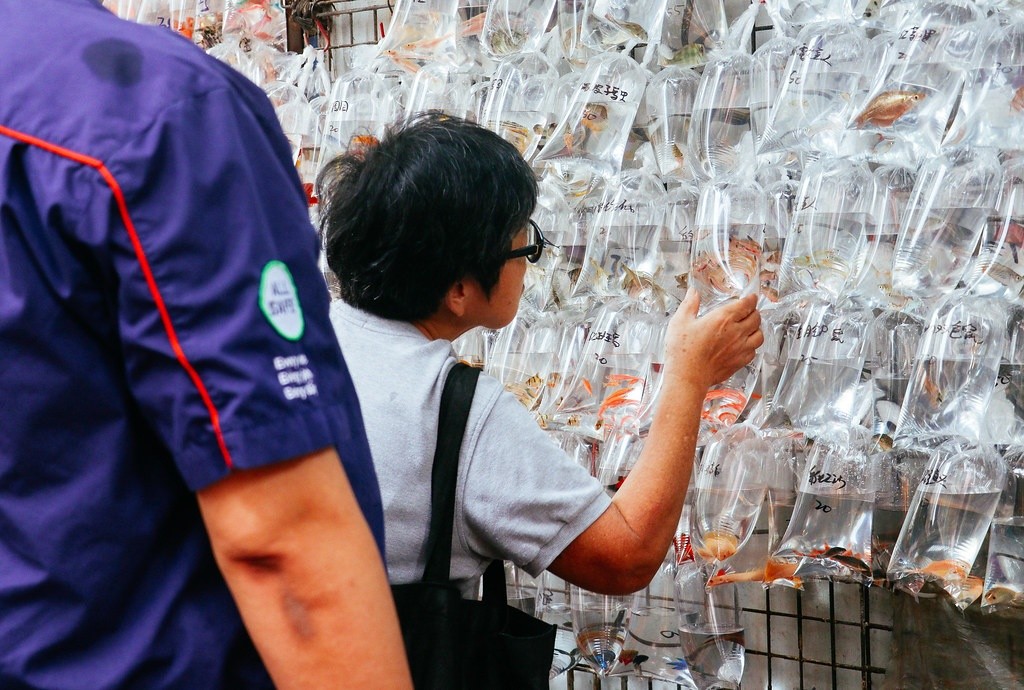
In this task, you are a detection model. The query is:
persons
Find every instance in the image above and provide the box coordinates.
[0,1,416,690]
[315,109,764,690]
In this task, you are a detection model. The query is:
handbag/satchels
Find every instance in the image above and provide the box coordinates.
[391,362,557,690]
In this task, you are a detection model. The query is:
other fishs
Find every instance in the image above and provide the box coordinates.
[173,1,1022,671]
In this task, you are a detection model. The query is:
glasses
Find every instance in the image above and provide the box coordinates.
[503,219,546,263]
